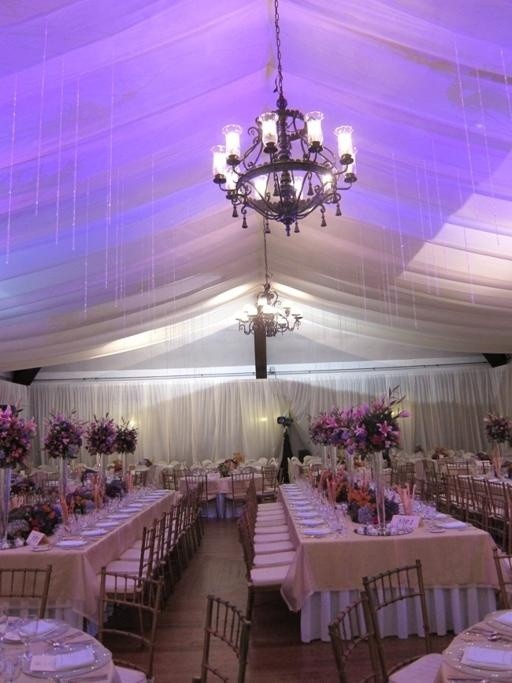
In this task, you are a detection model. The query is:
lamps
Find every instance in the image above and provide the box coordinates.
[237,223,303,337]
[211,0,357,236]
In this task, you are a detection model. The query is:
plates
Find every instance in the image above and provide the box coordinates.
[437,520,465,528]
[38,617,71,640]
[51,489,169,548]
[21,642,116,679]
[458,473,500,482]
[440,642,512,678]
[279,478,332,536]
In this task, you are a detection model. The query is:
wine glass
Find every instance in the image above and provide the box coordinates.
[14,612,38,655]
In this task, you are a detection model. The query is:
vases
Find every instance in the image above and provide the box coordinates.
[320,446,412,536]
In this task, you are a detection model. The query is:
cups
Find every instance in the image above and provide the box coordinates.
[419,499,437,532]
[333,500,349,538]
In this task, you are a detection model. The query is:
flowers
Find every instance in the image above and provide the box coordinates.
[307,384,410,461]
[0,402,139,468]
[483,411,512,446]
